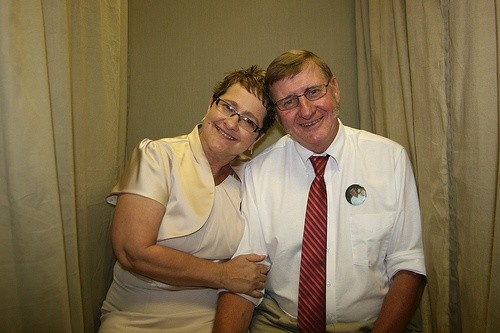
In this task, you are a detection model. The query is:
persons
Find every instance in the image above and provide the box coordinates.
[213,49,428,333]
[97,63,279,333]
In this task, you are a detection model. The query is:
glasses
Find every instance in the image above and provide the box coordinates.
[271,77,330,111]
[215,96,263,134]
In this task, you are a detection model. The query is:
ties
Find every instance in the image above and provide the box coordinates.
[296,154,330,333]
